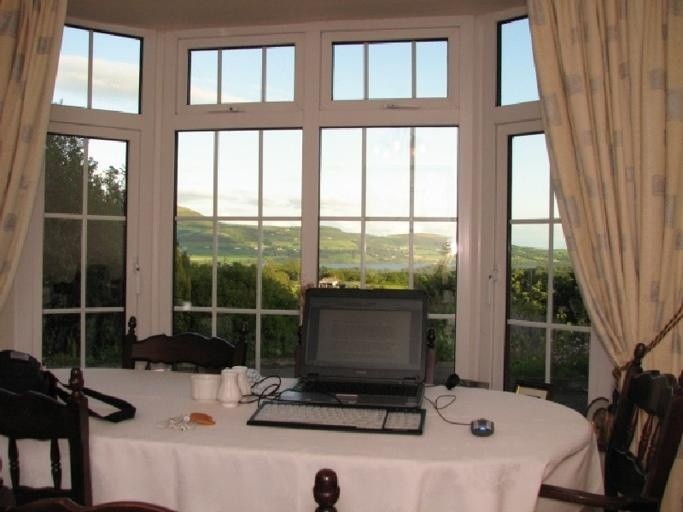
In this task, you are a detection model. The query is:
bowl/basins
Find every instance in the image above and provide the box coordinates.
[189,373,221,402]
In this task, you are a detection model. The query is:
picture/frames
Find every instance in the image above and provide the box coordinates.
[511,379,554,402]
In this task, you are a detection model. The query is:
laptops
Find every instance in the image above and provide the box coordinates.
[278,288,427,407]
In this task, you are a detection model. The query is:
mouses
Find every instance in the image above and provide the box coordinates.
[470,419,494,436]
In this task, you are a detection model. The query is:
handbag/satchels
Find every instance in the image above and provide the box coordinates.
[0,347,136,421]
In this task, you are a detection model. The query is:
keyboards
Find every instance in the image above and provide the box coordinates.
[246,401,427,434]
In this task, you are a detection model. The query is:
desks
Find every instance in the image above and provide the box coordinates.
[0,369,597,512]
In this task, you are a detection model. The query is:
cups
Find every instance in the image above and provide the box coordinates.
[216,364,253,409]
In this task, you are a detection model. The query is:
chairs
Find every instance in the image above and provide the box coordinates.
[539,339,683,512]
[112,314,251,374]
[0,346,94,511]
